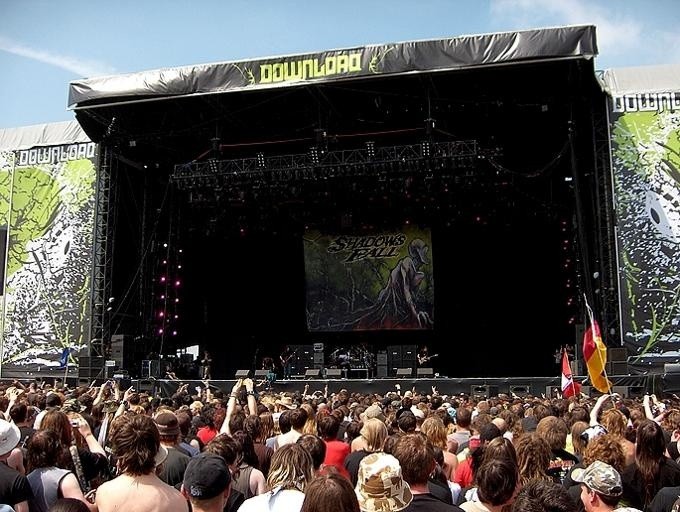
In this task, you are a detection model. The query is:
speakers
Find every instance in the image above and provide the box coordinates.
[546,386,560,400]
[613,386,628,399]
[575,324,628,375]
[509,384,530,398]
[471,385,498,404]
[79,335,132,378]
[305,352,342,379]
[580,385,595,397]
[255,370,270,380]
[235,369,253,379]
[0,226,7,296]
[376,345,433,377]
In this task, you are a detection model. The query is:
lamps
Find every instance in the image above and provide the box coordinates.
[208,81,438,162]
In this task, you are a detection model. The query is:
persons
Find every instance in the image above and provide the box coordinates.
[280,346,295,380]
[0,378,680,511]
[201,351,212,380]
[165,362,178,380]
[416,345,429,368]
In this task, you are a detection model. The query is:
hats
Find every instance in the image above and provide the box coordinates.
[274,397,298,410]
[58,398,88,414]
[154,409,179,436]
[101,399,121,414]
[404,390,413,400]
[359,404,385,423]
[153,442,169,467]
[0,418,21,455]
[271,413,283,435]
[571,460,623,497]
[522,416,538,433]
[354,452,413,512]
[183,453,231,500]
[441,402,451,409]
[4,387,24,400]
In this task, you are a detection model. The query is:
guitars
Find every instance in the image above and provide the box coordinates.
[281,347,299,367]
[419,354,438,365]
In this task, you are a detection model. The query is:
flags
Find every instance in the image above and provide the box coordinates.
[561,351,581,397]
[581,304,613,393]
